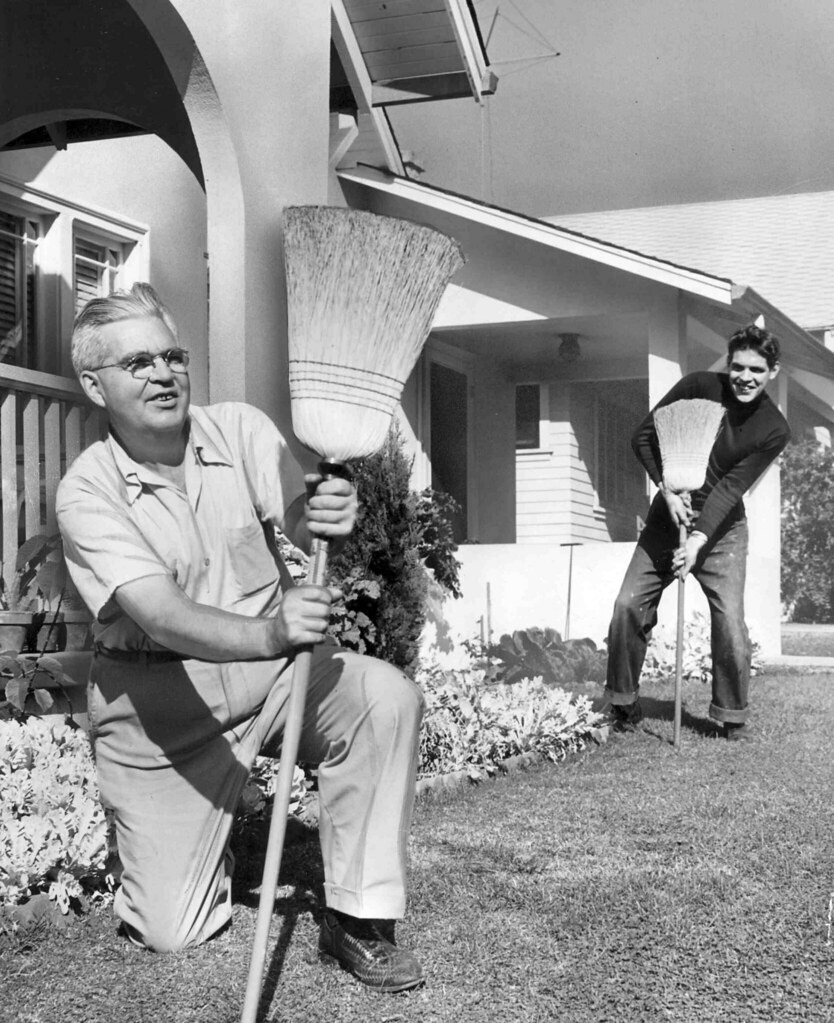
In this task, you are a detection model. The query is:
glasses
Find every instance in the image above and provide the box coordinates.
[92,348,189,379]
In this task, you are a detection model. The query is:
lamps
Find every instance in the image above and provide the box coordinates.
[558,333,581,362]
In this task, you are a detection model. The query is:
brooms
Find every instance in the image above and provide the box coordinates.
[238,202,473,1022]
[653,398,728,748]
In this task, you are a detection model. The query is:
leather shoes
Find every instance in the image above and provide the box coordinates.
[317,914,426,993]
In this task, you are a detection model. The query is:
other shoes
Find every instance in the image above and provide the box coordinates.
[614,704,642,733]
[723,722,745,741]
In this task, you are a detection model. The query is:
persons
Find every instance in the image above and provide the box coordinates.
[604,324,793,744]
[57,283,426,991]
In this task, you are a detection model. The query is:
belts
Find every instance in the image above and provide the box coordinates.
[96,644,191,663]
